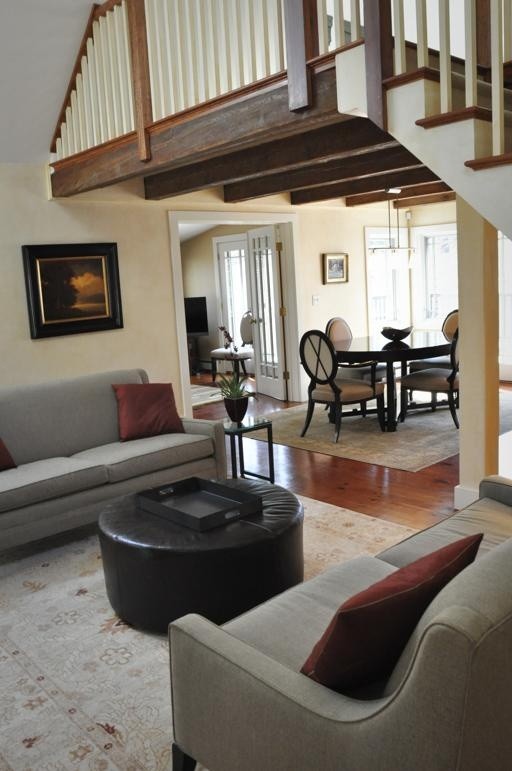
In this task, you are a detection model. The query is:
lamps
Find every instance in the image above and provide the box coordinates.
[369,187,418,268]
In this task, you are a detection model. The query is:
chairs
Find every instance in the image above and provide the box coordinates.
[210,310,253,382]
[300,309,459,445]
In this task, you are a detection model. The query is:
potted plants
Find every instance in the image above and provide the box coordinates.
[215,369,251,425]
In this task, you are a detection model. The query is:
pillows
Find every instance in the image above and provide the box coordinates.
[300,533,484,700]
[110,383,185,443]
[0,438,18,471]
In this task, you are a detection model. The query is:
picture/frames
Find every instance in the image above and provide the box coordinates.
[322,252,349,285]
[22,242,124,338]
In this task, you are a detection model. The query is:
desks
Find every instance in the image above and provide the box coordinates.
[97,479,303,631]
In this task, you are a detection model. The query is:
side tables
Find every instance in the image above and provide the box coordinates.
[213,414,275,482]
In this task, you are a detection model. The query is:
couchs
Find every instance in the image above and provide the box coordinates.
[0,368,227,553]
[169,476,511,770]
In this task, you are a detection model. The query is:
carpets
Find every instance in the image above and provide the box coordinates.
[191,382,256,410]
[242,386,511,474]
[1,493,423,770]
